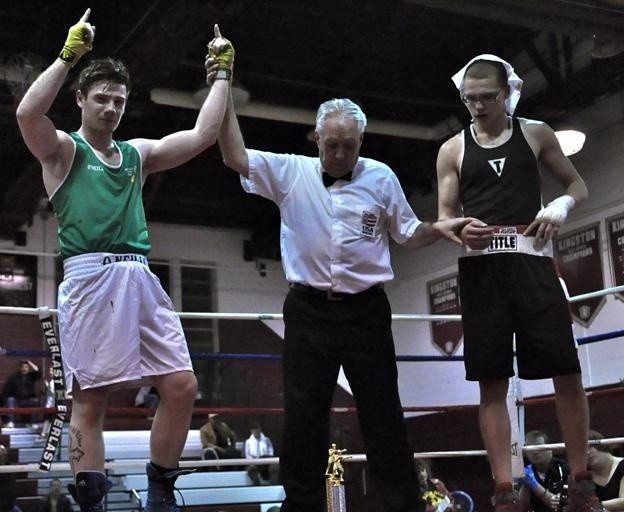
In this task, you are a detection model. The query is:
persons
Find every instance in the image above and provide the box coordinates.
[38,478,74,511]
[416,429,623,512]
[200,413,274,486]
[14,9,237,511]
[2,445,13,510]
[3,359,56,430]
[203,52,484,512]
[434,54,603,511]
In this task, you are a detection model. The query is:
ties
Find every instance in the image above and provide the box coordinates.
[322,171,352,188]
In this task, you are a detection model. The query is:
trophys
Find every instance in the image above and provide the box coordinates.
[324,444,353,511]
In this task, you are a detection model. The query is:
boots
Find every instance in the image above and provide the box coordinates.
[491,482,519,512]
[67,470,113,512]
[143,463,197,512]
[567,471,609,512]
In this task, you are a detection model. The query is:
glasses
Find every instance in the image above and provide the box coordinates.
[462,87,505,104]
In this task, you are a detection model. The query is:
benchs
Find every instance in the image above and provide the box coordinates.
[101,428,287,512]
